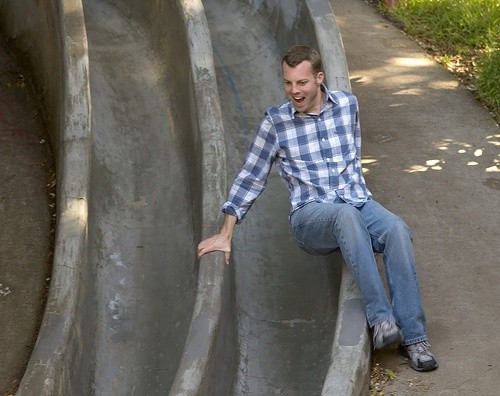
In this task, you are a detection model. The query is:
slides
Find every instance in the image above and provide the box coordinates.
[20,0,372,396]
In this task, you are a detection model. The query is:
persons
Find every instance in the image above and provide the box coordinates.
[197,44,440,372]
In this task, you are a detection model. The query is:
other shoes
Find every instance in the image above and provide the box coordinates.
[398,341,438,371]
[373,320,404,350]
